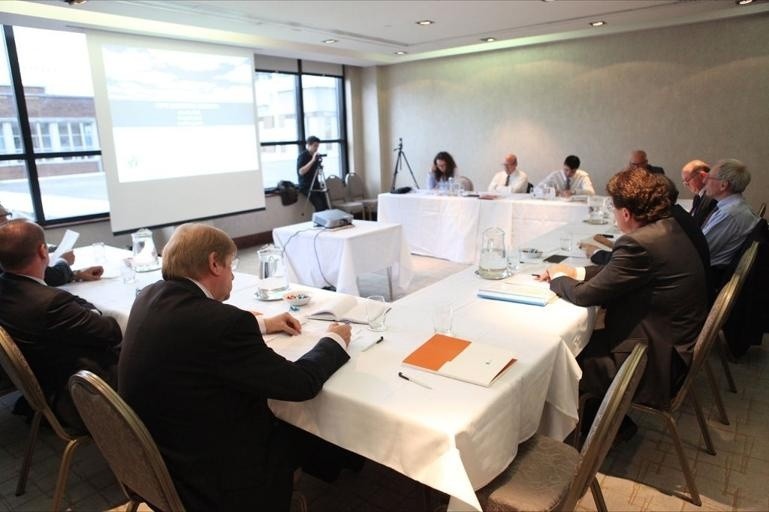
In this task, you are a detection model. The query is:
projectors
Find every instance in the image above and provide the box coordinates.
[312,208,353,228]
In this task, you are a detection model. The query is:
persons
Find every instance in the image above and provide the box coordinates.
[427,151,460,190]
[0,200,104,424]
[488,154,528,193]
[0,218,123,417]
[578,173,712,302]
[117,223,351,511]
[682,159,718,227]
[534,166,708,453]
[297,137,328,211]
[534,155,596,198]
[701,159,761,289]
[629,151,665,174]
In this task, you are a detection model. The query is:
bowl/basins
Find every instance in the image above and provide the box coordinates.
[519,249,543,257]
[283,291,310,306]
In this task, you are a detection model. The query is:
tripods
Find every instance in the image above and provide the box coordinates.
[391,150,419,190]
[303,166,331,209]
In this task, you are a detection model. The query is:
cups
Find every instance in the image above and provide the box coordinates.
[560,235,571,251]
[434,303,453,335]
[93,242,105,261]
[365,295,386,330]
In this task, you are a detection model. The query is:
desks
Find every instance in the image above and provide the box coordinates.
[45,189,694,512]
[273,220,405,301]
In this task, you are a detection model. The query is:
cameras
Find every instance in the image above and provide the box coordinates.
[315,153,327,162]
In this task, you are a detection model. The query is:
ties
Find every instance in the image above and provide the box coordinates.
[505,175,510,187]
[564,178,571,191]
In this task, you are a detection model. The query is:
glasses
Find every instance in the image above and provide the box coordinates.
[0,212,14,219]
[216,253,240,271]
[704,177,722,182]
[680,175,699,185]
[504,163,516,168]
[436,165,446,169]
[630,160,646,167]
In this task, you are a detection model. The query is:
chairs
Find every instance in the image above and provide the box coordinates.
[68,371,308,512]
[0,325,94,512]
[476,343,649,512]
[324,174,366,221]
[459,175,473,191]
[345,172,378,221]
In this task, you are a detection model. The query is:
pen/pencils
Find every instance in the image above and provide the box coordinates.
[363,336,386,352]
[398,371,434,391]
[532,274,539,277]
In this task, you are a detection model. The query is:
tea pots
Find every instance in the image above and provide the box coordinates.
[587,196,604,224]
[256,242,289,301]
[448,177,463,197]
[131,229,159,268]
[477,226,506,279]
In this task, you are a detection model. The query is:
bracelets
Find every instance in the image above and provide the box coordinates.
[73,270,81,282]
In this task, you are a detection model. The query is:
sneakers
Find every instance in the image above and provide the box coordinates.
[615,414,639,442]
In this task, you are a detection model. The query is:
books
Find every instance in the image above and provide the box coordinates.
[404,334,519,389]
[303,293,392,326]
[476,277,556,308]
